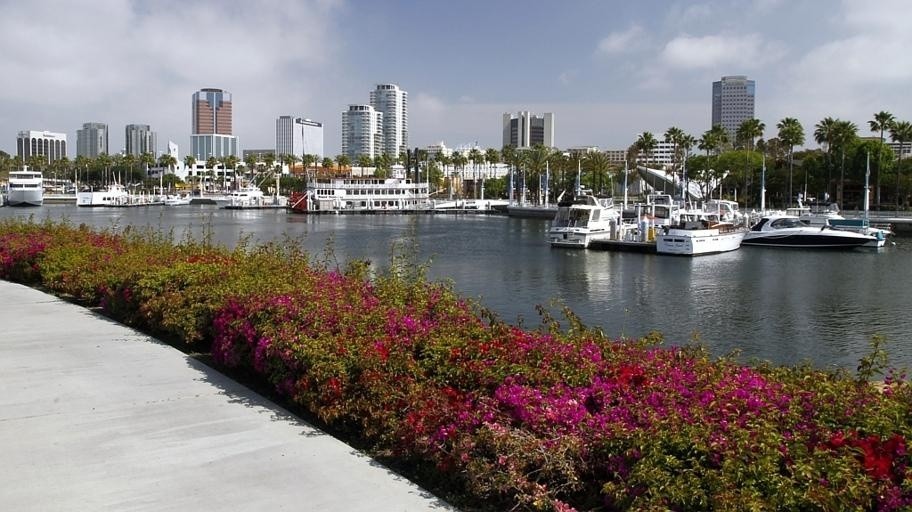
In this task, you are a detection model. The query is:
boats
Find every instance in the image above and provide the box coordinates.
[0,165,44,207]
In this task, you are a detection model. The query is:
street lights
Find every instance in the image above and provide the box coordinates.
[578,159,580,196]
[864,151,870,216]
[623,160,629,210]
[760,154,769,211]
[544,161,549,208]
